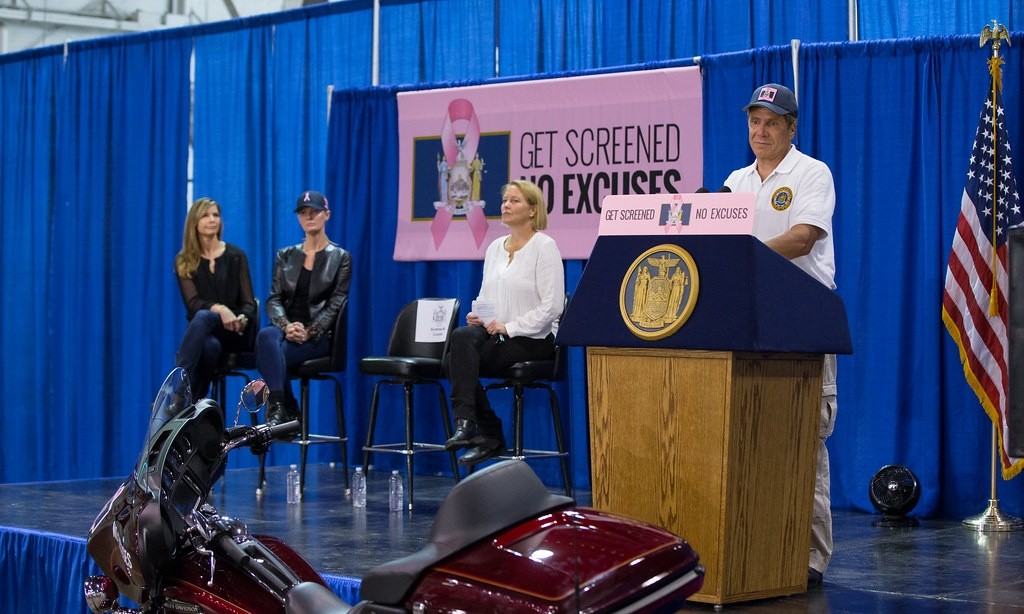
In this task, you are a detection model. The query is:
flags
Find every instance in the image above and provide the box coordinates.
[942,67,1024,481]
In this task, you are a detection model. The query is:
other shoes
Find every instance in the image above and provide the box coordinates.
[150,392,186,416]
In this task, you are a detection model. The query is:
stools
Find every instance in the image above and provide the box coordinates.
[361,298,461,519]
[466,294,574,509]
[202,351,268,496]
[256,298,351,503]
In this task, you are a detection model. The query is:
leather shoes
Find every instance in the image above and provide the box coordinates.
[445,418,484,451]
[264,400,302,442]
[457,432,506,464]
[808,566,824,589]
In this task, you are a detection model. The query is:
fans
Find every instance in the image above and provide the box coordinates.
[868,465,921,529]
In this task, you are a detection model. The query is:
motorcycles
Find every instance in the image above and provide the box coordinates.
[82,368,706,614]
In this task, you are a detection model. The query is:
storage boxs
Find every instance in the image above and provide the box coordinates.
[407,507,705,614]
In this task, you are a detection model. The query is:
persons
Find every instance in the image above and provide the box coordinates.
[256,190,353,442]
[723,83,837,592]
[445,179,565,465]
[164,197,257,417]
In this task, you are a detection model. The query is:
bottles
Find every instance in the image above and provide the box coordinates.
[352,468,366,507]
[389,471,403,511]
[287,465,300,503]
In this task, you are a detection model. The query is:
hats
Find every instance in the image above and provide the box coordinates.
[294,190,329,213]
[742,83,798,119]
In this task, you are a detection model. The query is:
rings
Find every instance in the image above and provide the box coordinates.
[490,329,493,331]
